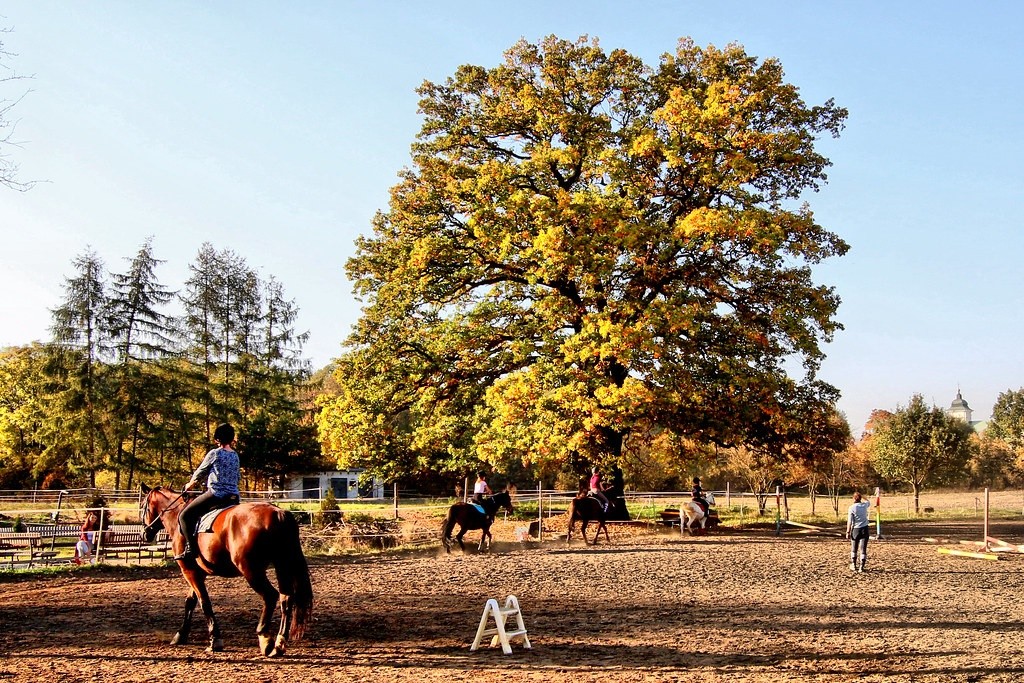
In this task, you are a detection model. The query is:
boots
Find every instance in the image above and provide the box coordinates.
[601,502,609,519]
[174,540,198,560]
[858,558,866,573]
[705,506,709,517]
[849,556,859,570]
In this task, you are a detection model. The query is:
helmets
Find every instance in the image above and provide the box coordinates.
[591,467,600,473]
[693,477,702,481]
[214,422,235,444]
[477,470,487,477]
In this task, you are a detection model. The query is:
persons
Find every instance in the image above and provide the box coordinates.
[474,471,494,522]
[845,492,871,572]
[75,512,99,566]
[590,467,609,514]
[690,477,710,518]
[173,423,240,560]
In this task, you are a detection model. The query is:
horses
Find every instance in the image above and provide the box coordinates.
[442,490,514,553]
[140,482,314,658]
[564,485,618,545]
[679,492,715,535]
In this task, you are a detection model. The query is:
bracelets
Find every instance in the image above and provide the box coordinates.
[846,531,850,534]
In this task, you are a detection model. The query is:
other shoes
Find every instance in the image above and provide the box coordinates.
[487,514,494,519]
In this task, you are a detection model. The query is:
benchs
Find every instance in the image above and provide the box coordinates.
[0,525,172,569]
